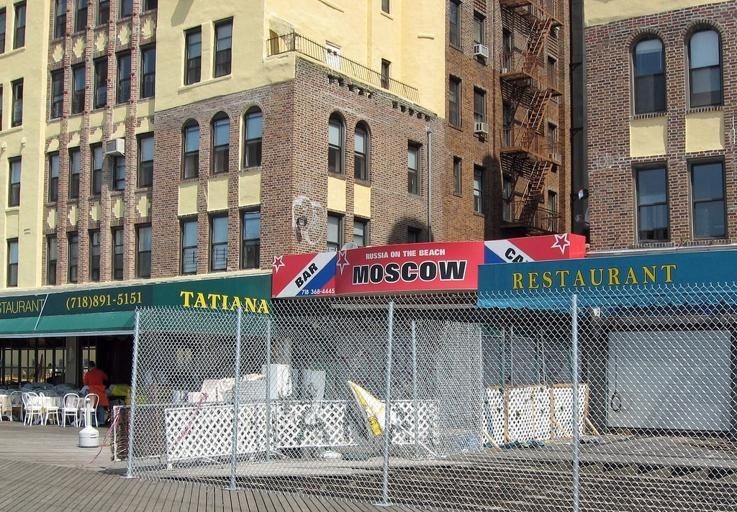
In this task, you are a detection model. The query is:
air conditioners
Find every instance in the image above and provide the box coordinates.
[104,137,124,159]
[474,121,490,137]
[474,44,489,60]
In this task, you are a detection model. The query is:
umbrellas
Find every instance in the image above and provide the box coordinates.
[348,381,437,457]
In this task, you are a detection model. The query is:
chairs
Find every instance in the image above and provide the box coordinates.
[0,383,100,429]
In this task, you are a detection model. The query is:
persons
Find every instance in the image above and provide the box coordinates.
[48,364,52,377]
[84,361,109,427]
